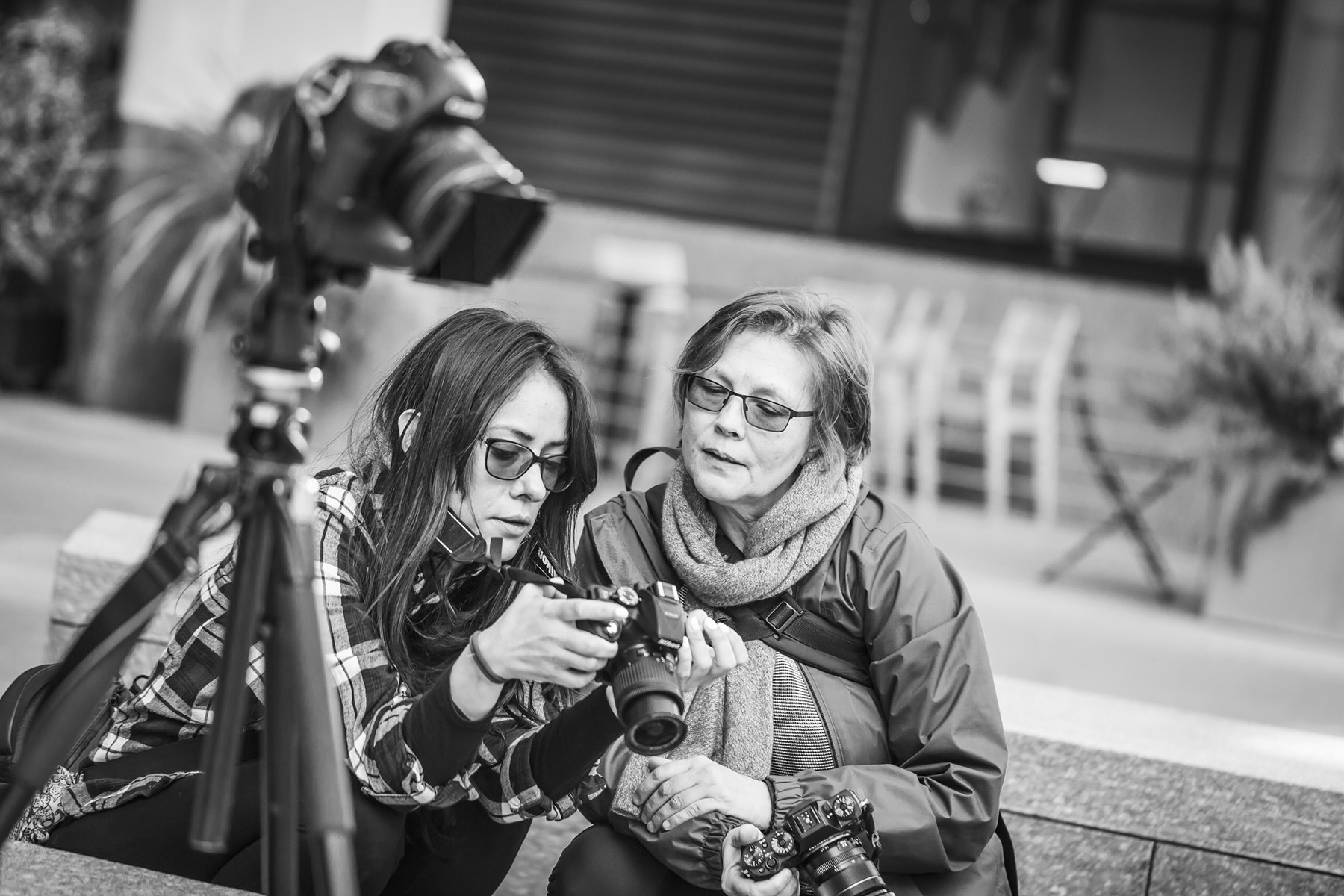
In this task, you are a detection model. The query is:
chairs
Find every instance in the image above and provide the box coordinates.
[953,304,1086,523]
[810,280,939,508]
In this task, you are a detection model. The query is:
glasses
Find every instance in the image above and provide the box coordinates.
[477,434,576,493]
[686,374,821,432]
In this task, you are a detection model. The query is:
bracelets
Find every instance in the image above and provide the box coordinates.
[469,629,507,685]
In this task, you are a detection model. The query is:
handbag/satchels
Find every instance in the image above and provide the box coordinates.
[0,661,135,802]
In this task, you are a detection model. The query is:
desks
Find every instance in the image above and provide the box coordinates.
[1037,339,1207,607]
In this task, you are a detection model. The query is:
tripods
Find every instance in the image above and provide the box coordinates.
[0,244,359,896]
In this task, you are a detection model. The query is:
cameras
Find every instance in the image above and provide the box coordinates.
[740,785,896,896]
[579,581,688,754]
[291,36,547,290]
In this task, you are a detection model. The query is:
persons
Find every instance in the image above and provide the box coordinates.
[548,288,1012,896]
[16,308,752,896]
